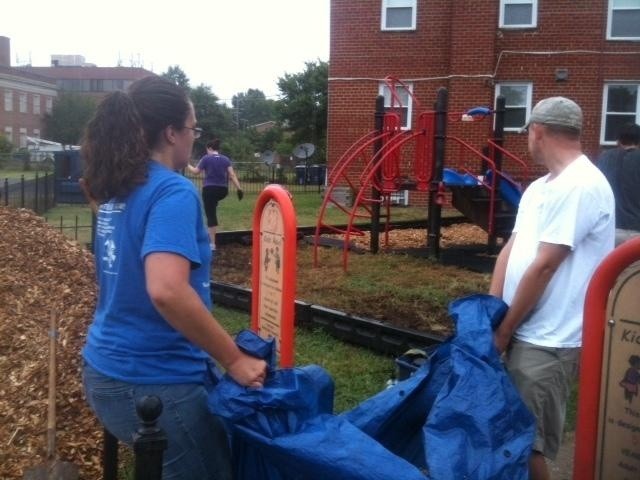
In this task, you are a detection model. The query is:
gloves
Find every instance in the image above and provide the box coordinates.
[236,189,244,202]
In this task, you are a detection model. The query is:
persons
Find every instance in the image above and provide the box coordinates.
[489,96,616,480]
[79,173,100,220]
[619,355,639,404]
[594,123,640,247]
[186,139,241,251]
[80,75,270,479]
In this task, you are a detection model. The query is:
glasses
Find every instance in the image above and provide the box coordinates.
[175,126,203,139]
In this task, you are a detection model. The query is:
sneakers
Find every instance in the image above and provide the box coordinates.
[210,244,218,251]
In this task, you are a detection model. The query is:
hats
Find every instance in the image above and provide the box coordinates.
[518,96,584,134]
